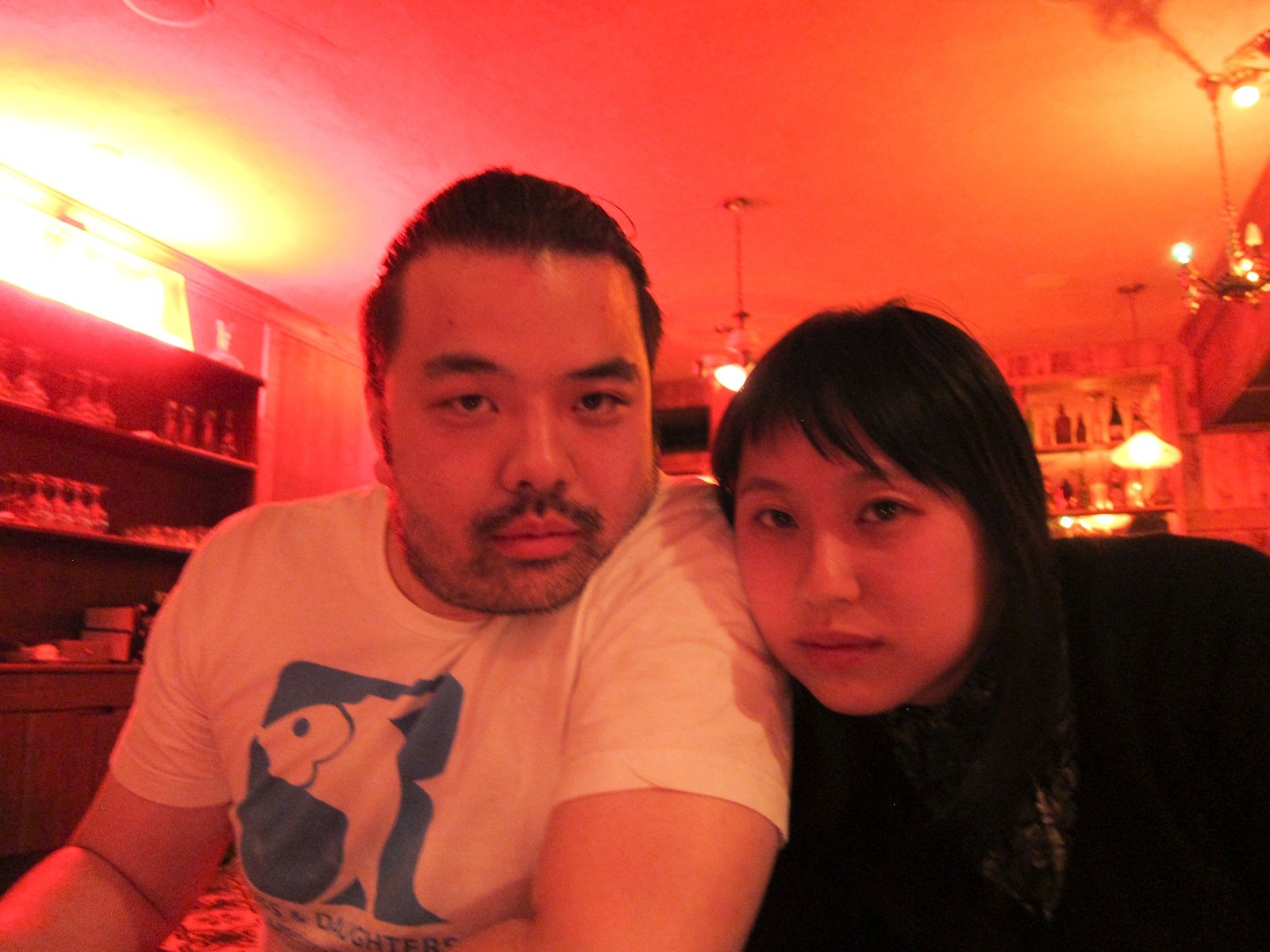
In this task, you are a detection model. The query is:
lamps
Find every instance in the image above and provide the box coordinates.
[1109,282,1183,471]
[690,198,757,393]
[1169,73,1270,314]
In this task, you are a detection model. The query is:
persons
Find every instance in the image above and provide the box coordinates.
[701,294,1270,950]
[1,163,795,952]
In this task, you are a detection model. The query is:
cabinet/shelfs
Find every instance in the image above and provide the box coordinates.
[0,660,142,859]
[0,283,268,669]
[1007,366,1188,538]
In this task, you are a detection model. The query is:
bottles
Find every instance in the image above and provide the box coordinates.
[1023,395,1143,511]
[149,397,240,459]
[11,344,115,435]
[3,468,111,542]
